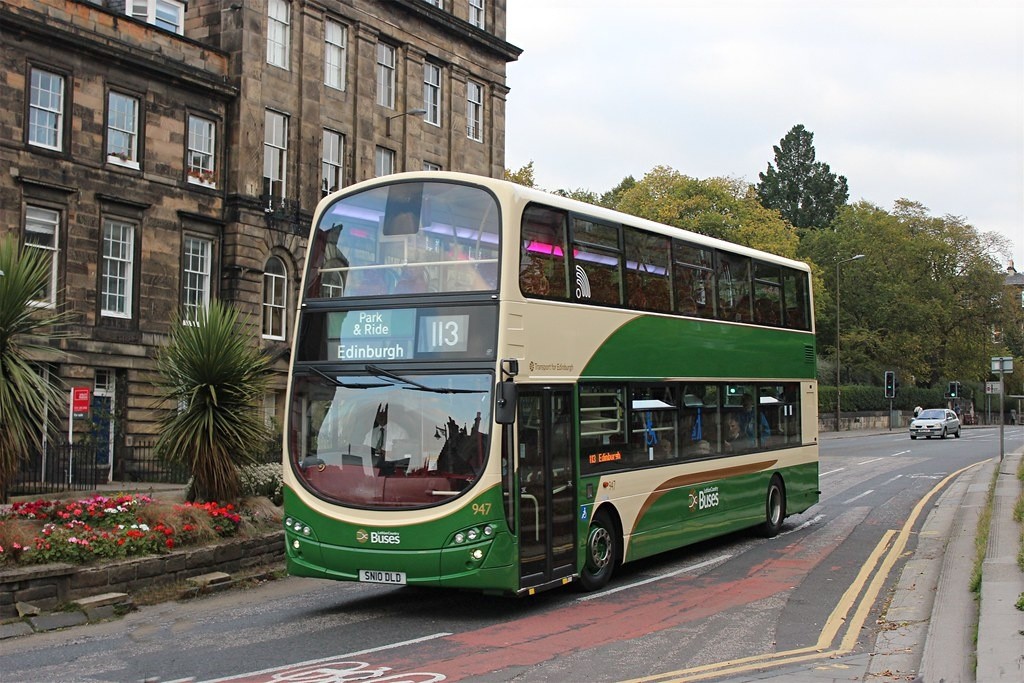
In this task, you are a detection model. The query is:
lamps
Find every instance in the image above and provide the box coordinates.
[385,109,427,136]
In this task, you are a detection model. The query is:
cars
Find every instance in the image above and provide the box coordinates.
[909,408,962,440]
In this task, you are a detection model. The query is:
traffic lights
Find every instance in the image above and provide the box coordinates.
[944,382,957,399]
[956,382,963,400]
[885,370,896,398]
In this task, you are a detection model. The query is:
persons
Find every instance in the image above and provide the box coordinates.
[357,261,389,294]
[724,415,751,452]
[735,394,771,447]
[914,404,924,418]
[1010,408,1017,425]
[608,434,622,444]
[658,439,672,460]
[953,403,960,419]
[362,403,409,451]
[695,440,711,454]
[393,265,426,294]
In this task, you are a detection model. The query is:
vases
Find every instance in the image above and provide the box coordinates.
[188,175,216,190]
[107,156,140,170]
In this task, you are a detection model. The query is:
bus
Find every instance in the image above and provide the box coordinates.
[280,170,823,596]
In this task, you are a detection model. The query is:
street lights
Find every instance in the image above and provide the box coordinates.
[835,254,866,432]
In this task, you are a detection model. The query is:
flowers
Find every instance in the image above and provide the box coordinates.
[187,166,217,185]
[107,151,132,161]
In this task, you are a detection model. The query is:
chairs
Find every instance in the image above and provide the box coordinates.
[522,254,801,328]
[634,403,794,459]
[354,255,499,295]
